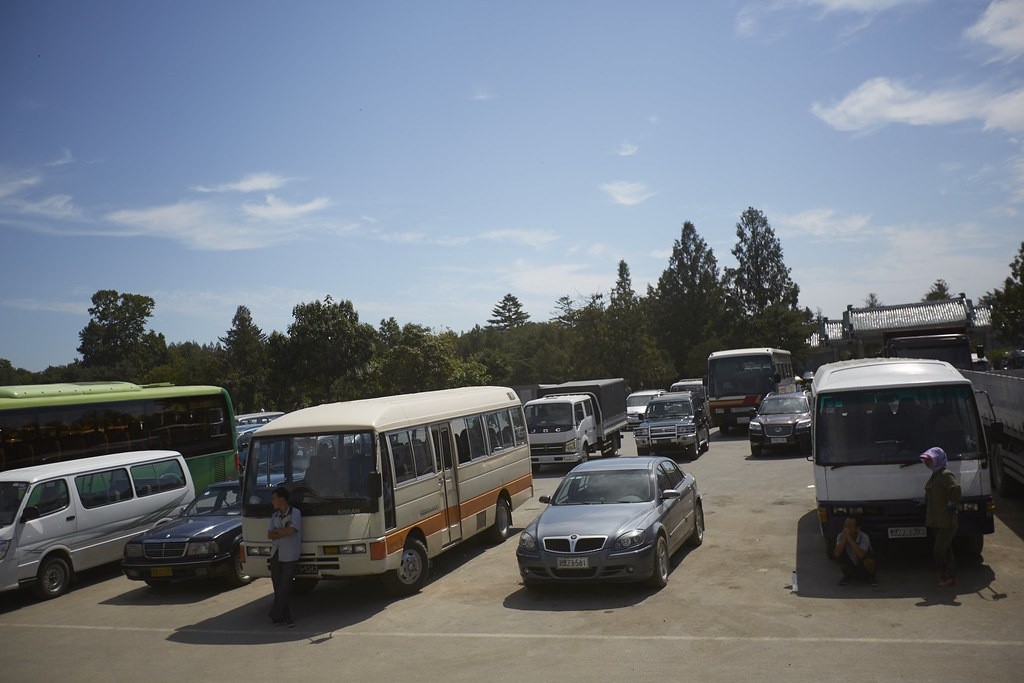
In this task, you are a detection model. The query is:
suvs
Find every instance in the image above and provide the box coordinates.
[623,386,668,430]
[745,387,811,457]
[634,391,711,461]
[668,376,711,422]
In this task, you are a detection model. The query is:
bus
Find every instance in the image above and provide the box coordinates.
[885,336,975,373]
[699,347,797,436]
[0,379,242,516]
[235,383,535,597]
[804,356,1002,568]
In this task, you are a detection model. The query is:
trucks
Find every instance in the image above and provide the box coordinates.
[523,377,630,474]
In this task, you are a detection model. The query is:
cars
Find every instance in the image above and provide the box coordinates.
[516,456,706,594]
[231,408,372,479]
[118,471,308,587]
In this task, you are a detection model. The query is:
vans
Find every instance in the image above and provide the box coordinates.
[0,450,198,598]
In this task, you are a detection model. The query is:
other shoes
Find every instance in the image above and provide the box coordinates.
[270,609,280,625]
[839,576,850,585]
[283,614,295,626]
[869,578,877,586]
[939,576,960,587]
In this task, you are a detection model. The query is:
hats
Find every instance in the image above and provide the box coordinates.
[919,454,932,459]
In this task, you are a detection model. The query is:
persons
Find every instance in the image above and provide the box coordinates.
[920,447,961,587]
[267,487,301,627]
[834,511,885,586]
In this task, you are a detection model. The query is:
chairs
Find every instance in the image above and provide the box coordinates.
[653,405,666,417]
[302,425,516,501]
[819,396,920,433]
[537,408,548,422]
[0,410,223,471]
[681,403,692,413]
[733,366,775,392]
[39,474,178,513]
